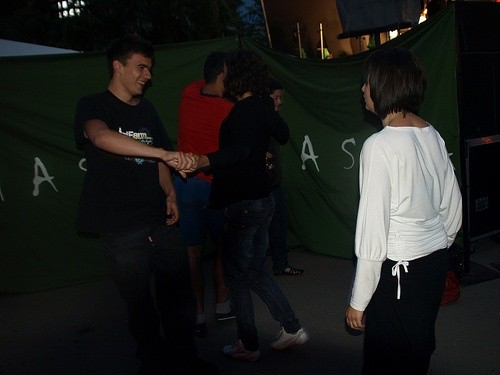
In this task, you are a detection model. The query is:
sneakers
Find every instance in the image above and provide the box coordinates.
[223,340,260,361]
[193,320,209,338]
[215,310,237,321]
[270,323,310,349]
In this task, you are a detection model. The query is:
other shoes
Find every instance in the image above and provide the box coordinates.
[273,265,304,276]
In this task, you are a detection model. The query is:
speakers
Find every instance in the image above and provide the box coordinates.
[426,0,500,243]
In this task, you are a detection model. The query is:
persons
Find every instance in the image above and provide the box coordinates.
[174,64,309,361]
[75,35,196,375]
[345,49,464,375]
[265,82,305,277]
[173,52,237,338]
